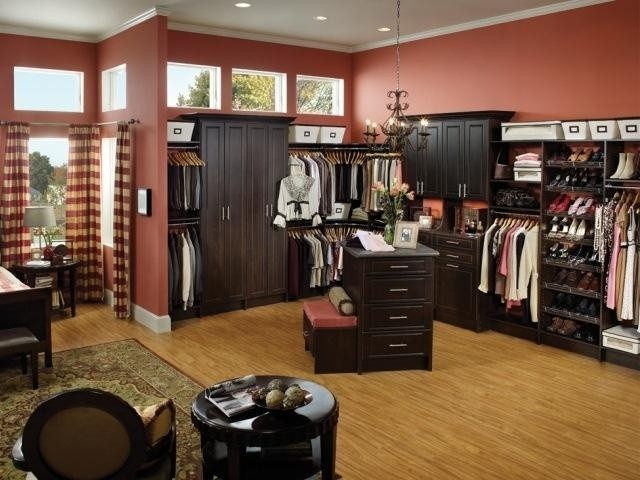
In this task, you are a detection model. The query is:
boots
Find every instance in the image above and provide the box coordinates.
[610,152,635,180]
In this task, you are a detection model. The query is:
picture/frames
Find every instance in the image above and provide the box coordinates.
[136,187,152,216]
[392,220,420,250]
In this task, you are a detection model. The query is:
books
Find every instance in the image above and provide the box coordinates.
[34,274,66,308]
[204,374,263,418]
[346,228,396,253]
[26,260,51,267]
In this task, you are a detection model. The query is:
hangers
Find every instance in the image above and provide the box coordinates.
[168,150,206,168]
[168,226,197,241]
[287,227,384,243]
[288,149,367,165]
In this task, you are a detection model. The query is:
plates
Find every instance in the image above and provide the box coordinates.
[248,387,316,414]
[55,245,69,256]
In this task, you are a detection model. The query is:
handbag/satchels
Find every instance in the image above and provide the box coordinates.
[493,164,514,180]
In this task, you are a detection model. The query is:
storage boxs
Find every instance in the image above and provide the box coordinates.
[317,124,347,144]
[498,116,640,139]
[166,119,196,142]
[288,123,320,144]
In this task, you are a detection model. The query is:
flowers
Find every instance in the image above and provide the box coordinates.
[370,177,416,240]
[32,224,60,246]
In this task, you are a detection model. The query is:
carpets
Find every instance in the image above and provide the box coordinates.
[0,337,347,480]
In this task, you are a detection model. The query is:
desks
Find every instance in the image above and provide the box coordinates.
[12,255,81,318]
[189,374,339,479]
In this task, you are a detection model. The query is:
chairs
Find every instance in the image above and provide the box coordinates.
[11,387,177,480]
[0,251,58,368]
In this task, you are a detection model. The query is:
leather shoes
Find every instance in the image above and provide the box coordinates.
[546,146,604,344]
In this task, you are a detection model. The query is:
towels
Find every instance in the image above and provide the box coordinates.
[25,260,51,268]
[35,274,65,309]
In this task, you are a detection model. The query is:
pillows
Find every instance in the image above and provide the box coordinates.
[127,398,177,448]
[328,285,356,316]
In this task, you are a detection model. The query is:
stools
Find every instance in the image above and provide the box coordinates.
[302,300,356,374]
[0,326,41,390]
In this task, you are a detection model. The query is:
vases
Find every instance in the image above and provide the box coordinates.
[384,220,395,245]
[43,247,54,260]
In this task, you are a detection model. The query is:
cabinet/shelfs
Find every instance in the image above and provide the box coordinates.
[401,114,442,198]
[288,142,399,300]
[418,228,434,247]
[339,238,441,373]
[434,230,491,333]
[200,112,245,316]
[442,110,518,200]
[244,113,297,311]
[492,140,640,374]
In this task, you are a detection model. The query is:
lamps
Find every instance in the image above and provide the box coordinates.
[23,206,57,266]
[361,2,432,154]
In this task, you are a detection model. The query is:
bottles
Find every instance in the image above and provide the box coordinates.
[477,221,484,234]
[468,221,475,233]
